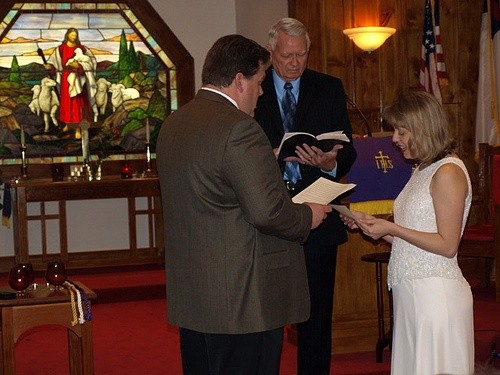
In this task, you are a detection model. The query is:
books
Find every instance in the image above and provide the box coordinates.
[277,132,351,164]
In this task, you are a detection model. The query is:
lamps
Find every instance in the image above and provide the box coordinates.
[343,27,396,53]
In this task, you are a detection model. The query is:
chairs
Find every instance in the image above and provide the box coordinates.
[458,144,500,302]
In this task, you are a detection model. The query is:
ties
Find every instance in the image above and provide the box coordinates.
[281,83,302,181]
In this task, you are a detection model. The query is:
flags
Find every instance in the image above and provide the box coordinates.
[471,0,500,213]
[418,0,451,107]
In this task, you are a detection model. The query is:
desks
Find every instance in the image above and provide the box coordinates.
[0,280,98,375]
[8,172,165,271]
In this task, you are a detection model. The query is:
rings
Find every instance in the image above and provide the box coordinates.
[308,157,312,161]
[305,162,306,165]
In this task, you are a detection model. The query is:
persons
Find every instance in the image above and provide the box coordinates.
[251,18,356,375]
[156,35,333,375]
[340,89,475,375]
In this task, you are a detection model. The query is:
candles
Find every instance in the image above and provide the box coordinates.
[146,117,150,143]
[20,125,26,146]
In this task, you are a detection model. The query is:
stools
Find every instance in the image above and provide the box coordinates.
[362,252,393,363]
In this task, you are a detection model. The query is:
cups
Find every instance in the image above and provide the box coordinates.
[52,165,64,181]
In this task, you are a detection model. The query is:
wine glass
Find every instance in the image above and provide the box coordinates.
[8,262,34,300]
[46,262,66,297]
